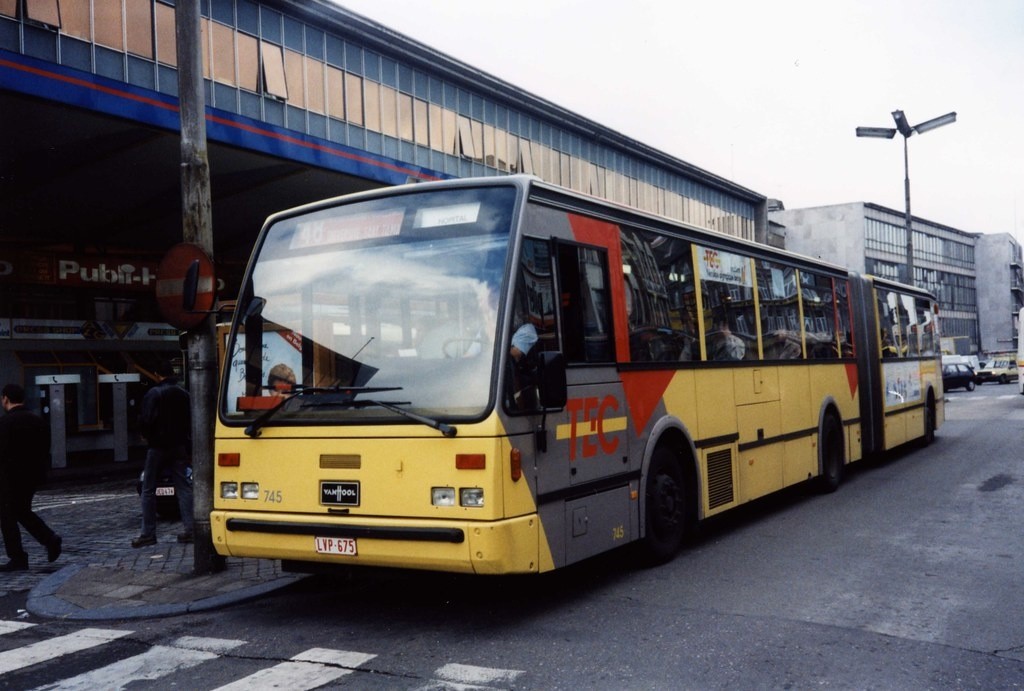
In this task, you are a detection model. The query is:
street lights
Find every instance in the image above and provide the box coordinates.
[856,109,957,287]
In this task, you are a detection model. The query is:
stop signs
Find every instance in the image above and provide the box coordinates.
[154,243,216,331]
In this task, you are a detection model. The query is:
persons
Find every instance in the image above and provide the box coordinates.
[882,324,932,357]
[467,280,540,406]
[268,364,296,398]
[710,310,745,360]
[0,384,63,573]
[131,360,194,548]
[674,305,699,361]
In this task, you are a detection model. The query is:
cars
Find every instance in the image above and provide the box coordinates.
[942,357,1018,392]
[138,449,214,521]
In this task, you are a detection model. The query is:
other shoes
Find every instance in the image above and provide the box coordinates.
[176,530,194,544]
[131,533,158,548]
[0,551,29,572]
[46,534,63,563]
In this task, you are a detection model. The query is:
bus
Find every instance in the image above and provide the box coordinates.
[210,174,944,574]
